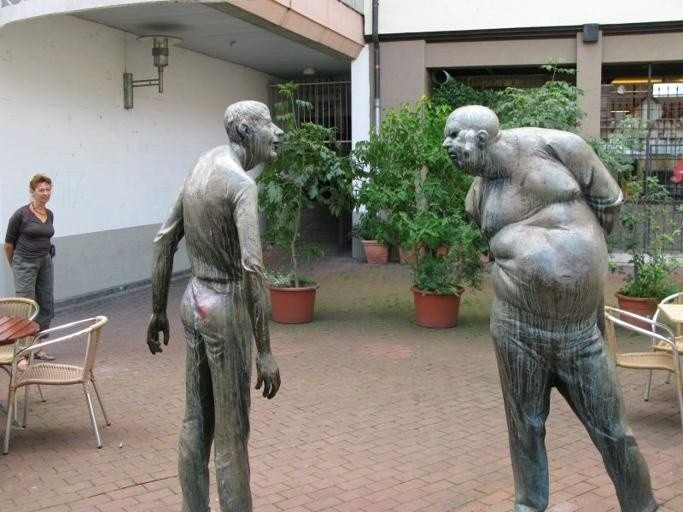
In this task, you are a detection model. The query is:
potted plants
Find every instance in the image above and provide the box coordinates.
[348,94,495,330]
[593,114,683,330]
[259,76,361,325]
[349,212,390,266]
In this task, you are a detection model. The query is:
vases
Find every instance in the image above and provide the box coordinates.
[476,251,489,263]
[396,239,420,267]
[434,243,449,258]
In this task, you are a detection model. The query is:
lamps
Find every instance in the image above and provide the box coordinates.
[120,31,186,111]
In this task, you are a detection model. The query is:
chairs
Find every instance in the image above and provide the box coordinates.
[0,296,49,432]
[600,302,681,420]
[2,313,114,456]
[642,290,682,411]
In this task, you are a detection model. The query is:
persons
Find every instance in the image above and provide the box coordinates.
[439,104,659,512]
[3,174,58,371]
[143,98,287,511]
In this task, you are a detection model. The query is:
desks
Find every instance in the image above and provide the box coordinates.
[657,304,682,337]
[0,313,40,436]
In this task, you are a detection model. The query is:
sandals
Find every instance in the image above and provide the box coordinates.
[33,350,57,362]
[17,356,28,373]
